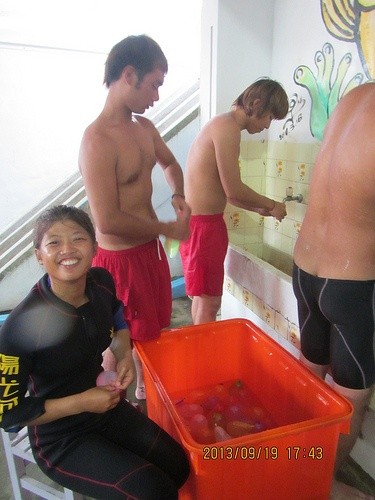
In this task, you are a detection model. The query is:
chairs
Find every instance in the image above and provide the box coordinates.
[0,424,75,500]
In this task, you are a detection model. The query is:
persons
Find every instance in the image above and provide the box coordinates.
[179,79,289,325]
[79,35,191,400]
[292,82,375,500]
[0,205,191,500]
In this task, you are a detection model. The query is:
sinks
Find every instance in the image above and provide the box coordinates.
[227,237,294,278]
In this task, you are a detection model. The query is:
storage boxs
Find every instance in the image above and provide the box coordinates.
[131,317,355,500]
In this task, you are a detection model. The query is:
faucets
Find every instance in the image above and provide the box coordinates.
[283,187,300,204]
